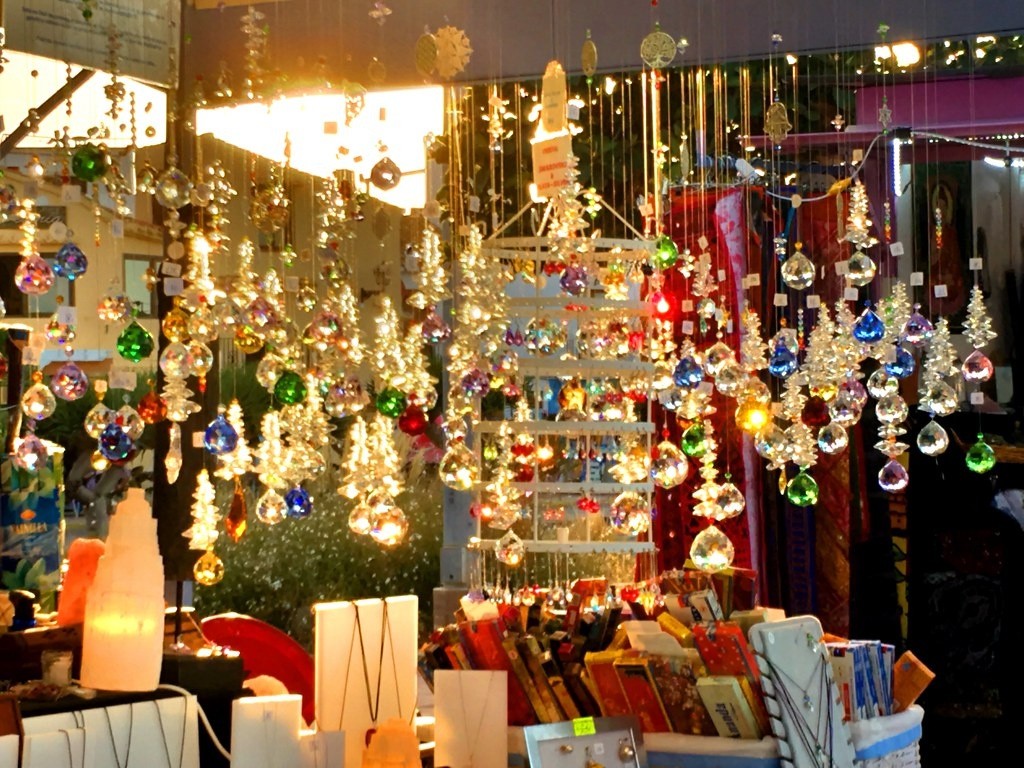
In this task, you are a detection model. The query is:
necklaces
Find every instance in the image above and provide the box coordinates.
[752,641,841,768]
[312,598,423,736]
[456,670,494,765]
[59,694,188,768]
